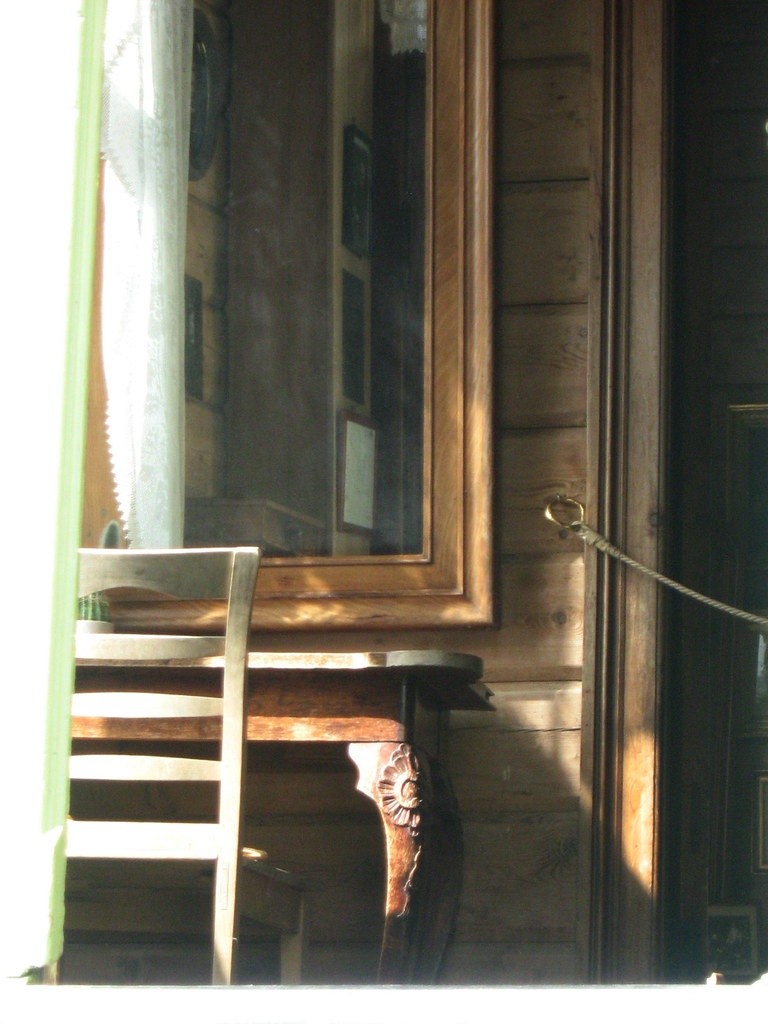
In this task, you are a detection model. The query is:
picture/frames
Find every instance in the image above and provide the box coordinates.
[344,124,373,255]
[706,902,764,980]
[750,769,768,876]
[184,274,202,403]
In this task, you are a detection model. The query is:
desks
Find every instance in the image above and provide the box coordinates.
[61,650,496,983]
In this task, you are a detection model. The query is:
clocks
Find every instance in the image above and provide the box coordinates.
[187,9,221,183]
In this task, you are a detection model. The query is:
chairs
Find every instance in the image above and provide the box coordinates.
[48,546,329,986]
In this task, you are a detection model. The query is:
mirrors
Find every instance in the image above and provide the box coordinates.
[76,0,496,634]
